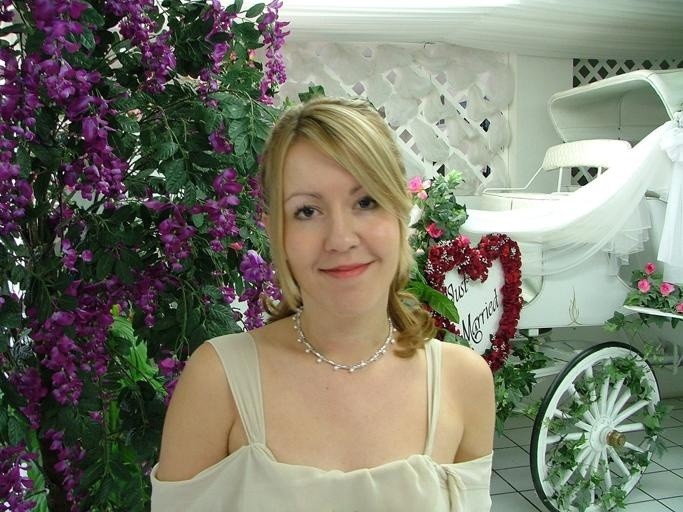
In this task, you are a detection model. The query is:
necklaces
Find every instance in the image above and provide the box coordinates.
[292,305,397,375]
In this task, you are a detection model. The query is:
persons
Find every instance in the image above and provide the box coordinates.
[148,96,497,511]
